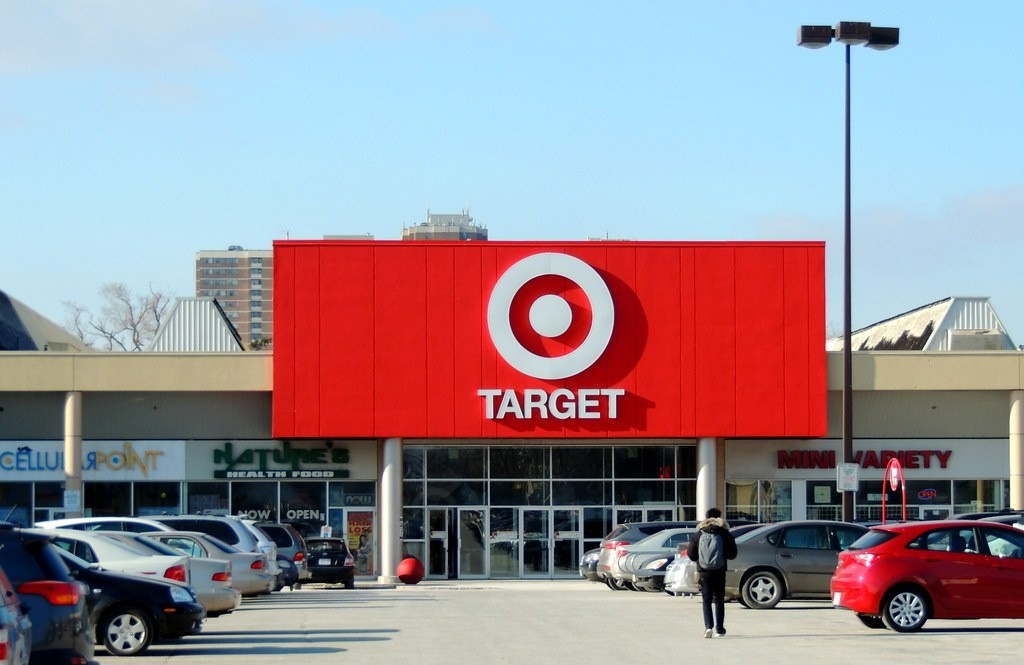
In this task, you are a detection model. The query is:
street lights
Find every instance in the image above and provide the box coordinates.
[793,20,901,522]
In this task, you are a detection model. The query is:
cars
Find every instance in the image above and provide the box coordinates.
[824,520,1024,632]
[682,520,870,611]
[291,535,356,589]
[1,504,315,665]
[911,507,1024,557]
[579,521,777,598]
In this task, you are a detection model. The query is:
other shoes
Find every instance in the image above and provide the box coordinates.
[704,628,713,638]
[715,633,725,636]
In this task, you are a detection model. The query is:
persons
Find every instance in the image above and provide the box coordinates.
[348,525,373,574]
[687,508,738,638]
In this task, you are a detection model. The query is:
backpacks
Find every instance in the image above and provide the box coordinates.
[699,529,723,568]
